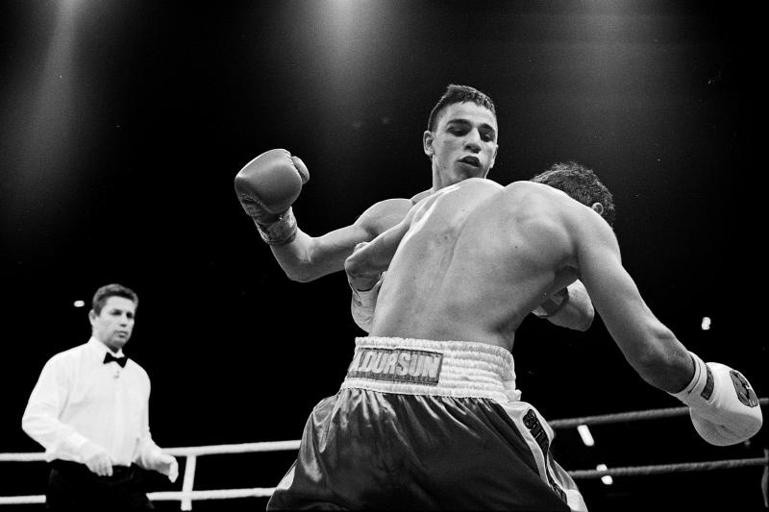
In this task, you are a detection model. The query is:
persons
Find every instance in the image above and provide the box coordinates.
[264,158,765,511]
[20,283,181,510]
[233,80,598,512]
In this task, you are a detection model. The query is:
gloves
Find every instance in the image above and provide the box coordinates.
[154,453,179,484]
[666,350,763,447]
[531,287,569,318]
[348,272,384,334]
[234,148,311,246]
[85,449,114,477]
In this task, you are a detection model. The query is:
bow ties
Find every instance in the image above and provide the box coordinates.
[102,352,131,368]
